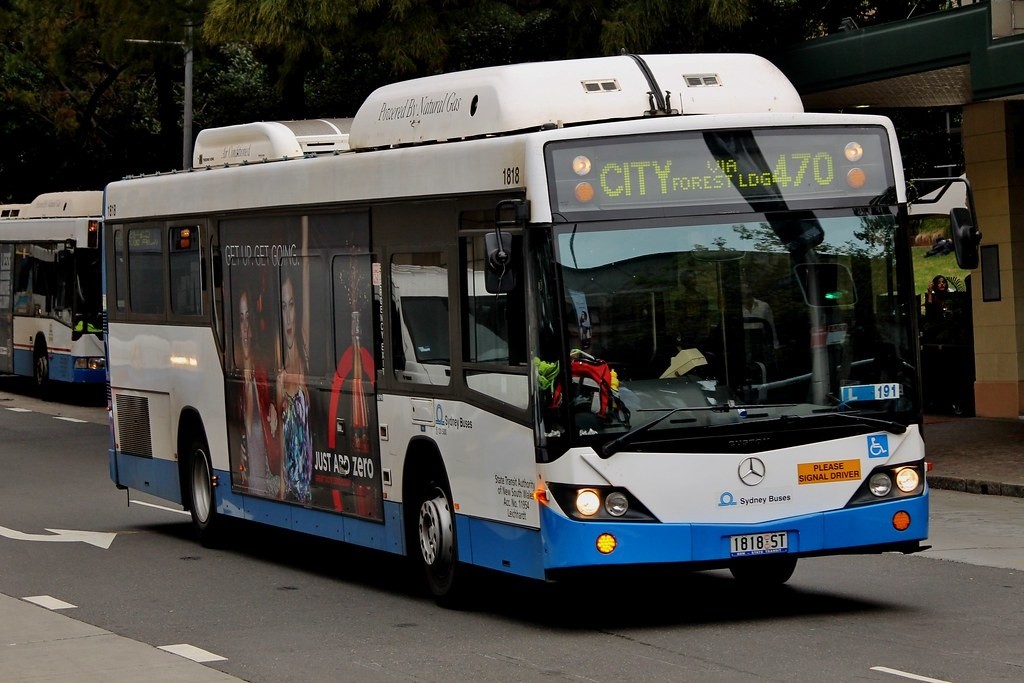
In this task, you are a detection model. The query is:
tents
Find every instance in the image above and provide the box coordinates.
[907,171,968,219]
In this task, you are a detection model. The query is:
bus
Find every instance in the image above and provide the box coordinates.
[97,51,983,605]
[0,188,221,409]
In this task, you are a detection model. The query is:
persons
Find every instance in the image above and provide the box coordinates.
[233,275,312,507]
[926,275,955,315]
[741,281,780,362]
[936,234,945,244]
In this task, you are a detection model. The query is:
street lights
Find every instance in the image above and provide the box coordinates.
[124,21,195,170]
